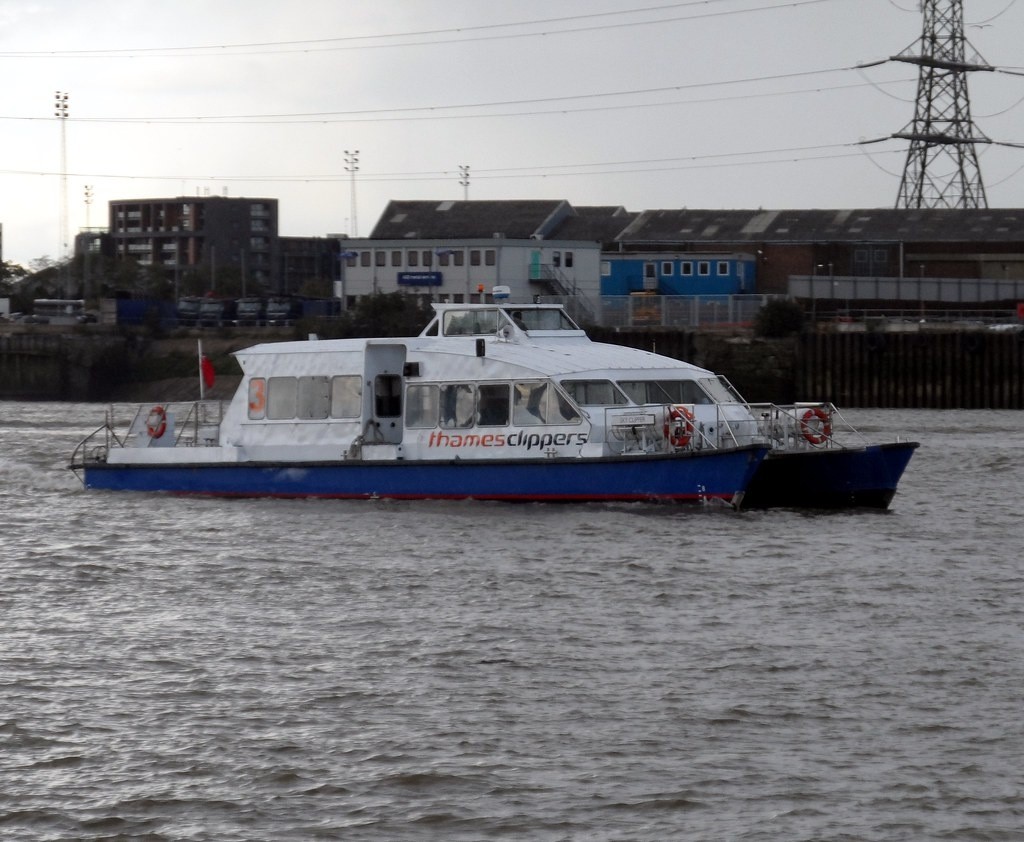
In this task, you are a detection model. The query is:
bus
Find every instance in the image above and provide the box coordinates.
[30,297,88,327]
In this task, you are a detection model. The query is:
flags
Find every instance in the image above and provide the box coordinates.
[201,355,216,389]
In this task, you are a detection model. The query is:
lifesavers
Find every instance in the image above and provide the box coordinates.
[147,405,167,439]
[664,406,694,447]
[801,409,831,445]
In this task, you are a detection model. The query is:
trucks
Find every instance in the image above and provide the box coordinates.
[176,294,298,329]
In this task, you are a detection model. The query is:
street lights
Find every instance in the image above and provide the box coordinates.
[811,263,825,325]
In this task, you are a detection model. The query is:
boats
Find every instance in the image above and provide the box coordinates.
[66,281,778,512]
[737,399,923,514]
